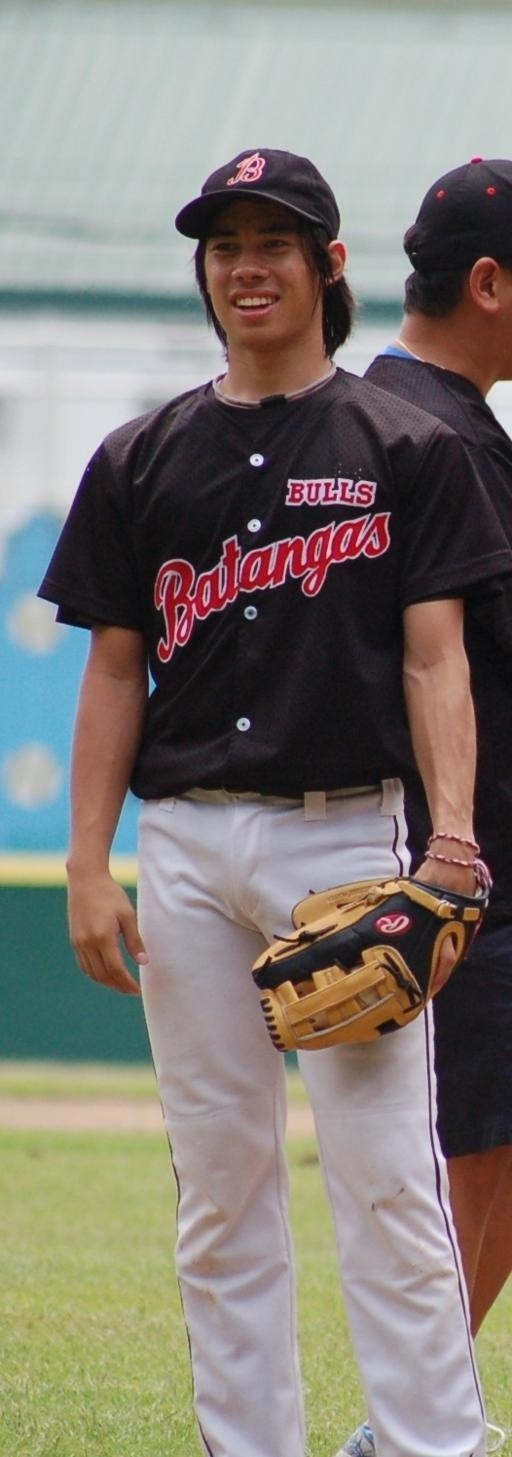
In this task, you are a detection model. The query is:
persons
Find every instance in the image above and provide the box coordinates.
[333,156,512,1457]
[32,148,512,1457]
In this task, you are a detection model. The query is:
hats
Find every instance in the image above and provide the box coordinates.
[175,148,341,239]
[403,156,512,270]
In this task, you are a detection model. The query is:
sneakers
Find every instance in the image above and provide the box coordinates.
[333,1418,377,1457]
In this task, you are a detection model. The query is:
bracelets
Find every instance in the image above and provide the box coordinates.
[422,831,493,890]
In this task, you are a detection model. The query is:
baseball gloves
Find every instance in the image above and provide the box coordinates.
[253,876,491,1051]
